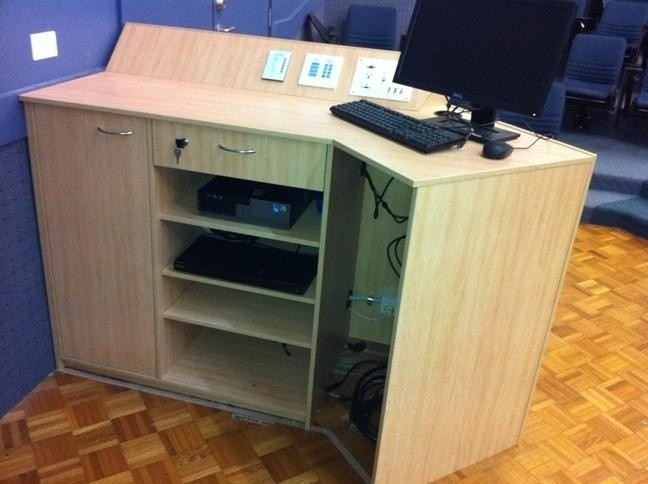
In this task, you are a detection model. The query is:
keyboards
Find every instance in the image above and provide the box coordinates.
[328,99,466,155]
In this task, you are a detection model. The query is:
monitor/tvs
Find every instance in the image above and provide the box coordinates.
[392,0,580,145]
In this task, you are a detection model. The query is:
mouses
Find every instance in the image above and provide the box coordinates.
[481,141,513,160]
